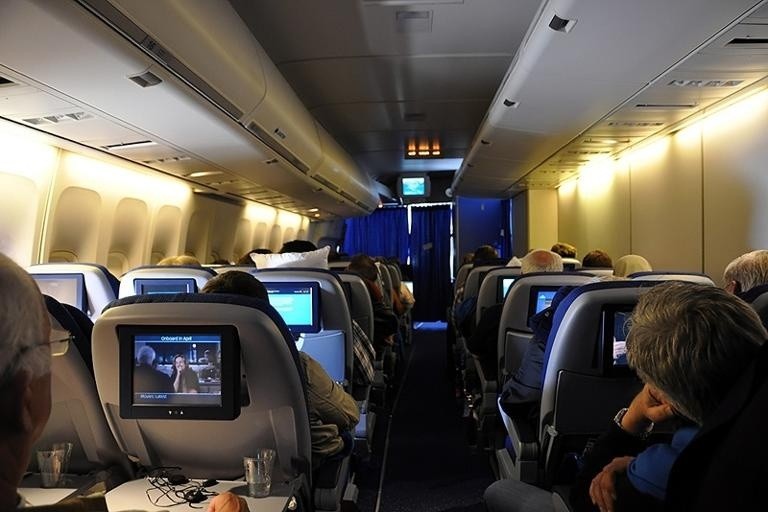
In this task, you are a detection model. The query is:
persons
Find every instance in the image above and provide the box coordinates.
[157,237,404,412]
[447,242,768,510]
[201,271,359,459]
[135,346,200,394]
[0,253,249,511]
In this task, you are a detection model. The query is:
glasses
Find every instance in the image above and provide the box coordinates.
[3,326,76,382]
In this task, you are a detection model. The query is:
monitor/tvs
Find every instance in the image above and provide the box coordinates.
[398,280,414,297]
[251,281,319,333]
[118,325,238,419]
[601,308,635,366]
[133,277,196,302]
[497,275,515,295]
[527,284,561,330]
[399,175,428,199]
[32,274,84,312]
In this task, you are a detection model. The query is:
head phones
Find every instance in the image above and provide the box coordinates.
[335,239,344,255]
[169,474,203,503]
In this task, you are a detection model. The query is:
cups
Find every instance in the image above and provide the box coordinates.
[35,440,76,486]
[241,448,278,498]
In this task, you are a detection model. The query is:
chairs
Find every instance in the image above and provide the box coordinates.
[0,246,417,512]
[452,256,767,512]
